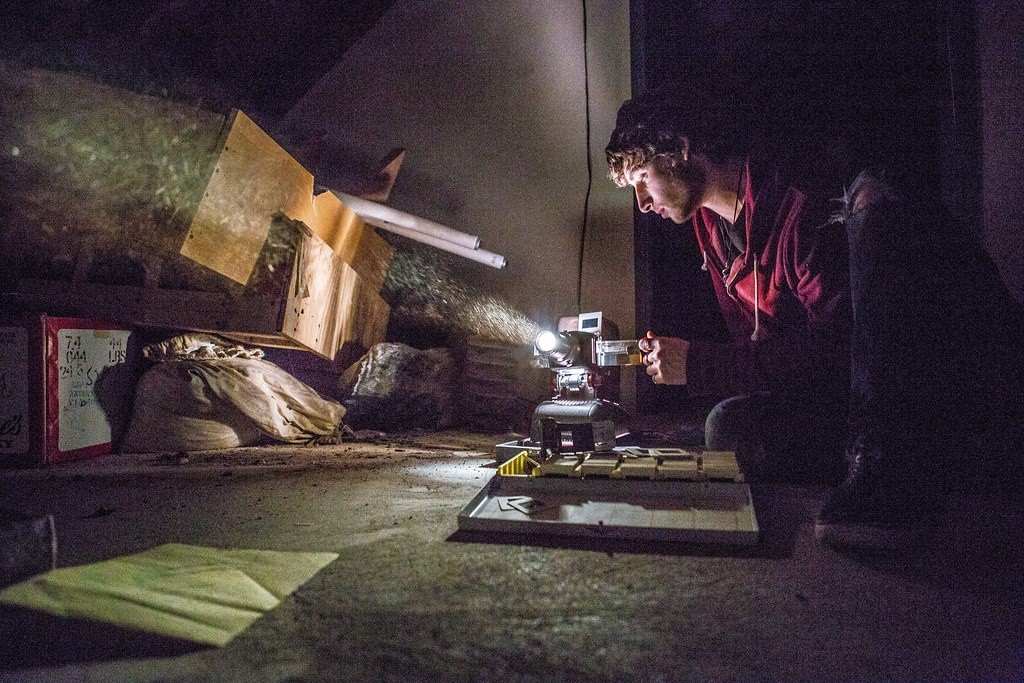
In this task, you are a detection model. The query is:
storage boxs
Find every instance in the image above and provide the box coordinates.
[0,309,133,467]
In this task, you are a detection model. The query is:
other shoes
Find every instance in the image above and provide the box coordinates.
[815,453,915,543]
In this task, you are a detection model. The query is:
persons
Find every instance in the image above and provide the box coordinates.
[604,91,1024,546]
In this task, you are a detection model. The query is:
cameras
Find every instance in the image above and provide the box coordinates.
[533,312,642,398]
